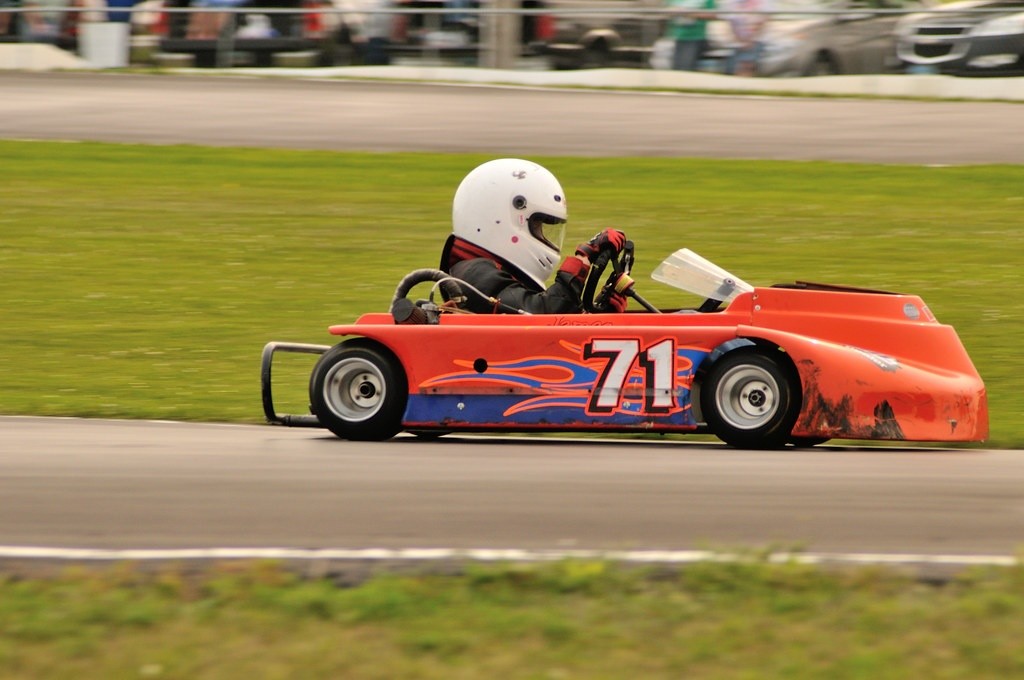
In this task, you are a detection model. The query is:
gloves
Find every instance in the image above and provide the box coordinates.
[574,226,628,265]
[593,282,628,314]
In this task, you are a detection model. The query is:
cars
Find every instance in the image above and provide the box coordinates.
[751,0,916,80]
[889,0,1023,79]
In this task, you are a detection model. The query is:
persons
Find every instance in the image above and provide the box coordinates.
[435,153,631,316]
[0,0,568,65]
[723,0,769,80]
[663,2,723,73]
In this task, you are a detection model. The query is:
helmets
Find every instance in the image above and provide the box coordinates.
[450,156,567,290]
